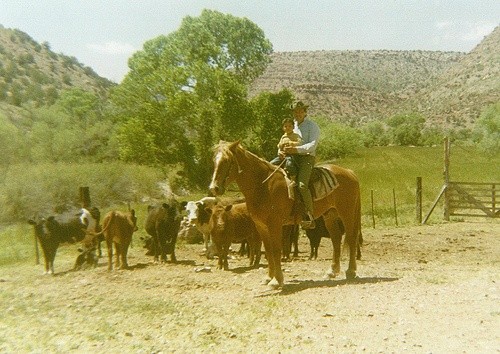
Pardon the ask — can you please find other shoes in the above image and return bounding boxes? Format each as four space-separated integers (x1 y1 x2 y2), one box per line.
290 176 297 186
302 214 315 229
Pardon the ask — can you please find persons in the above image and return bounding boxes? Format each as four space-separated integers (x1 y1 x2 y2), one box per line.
277 100 320 230
269 118 302 188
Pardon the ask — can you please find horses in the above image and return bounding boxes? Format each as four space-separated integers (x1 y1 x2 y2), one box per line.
209 138 363 286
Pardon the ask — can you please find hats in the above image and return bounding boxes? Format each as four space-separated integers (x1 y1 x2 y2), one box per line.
290 101 309 109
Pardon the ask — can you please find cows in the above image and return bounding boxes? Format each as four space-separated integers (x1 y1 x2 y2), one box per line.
282 207 363 262
84 209 138 270
185 197 232 252
27 206 100 275
204 201 262 271
146 198 188 264
